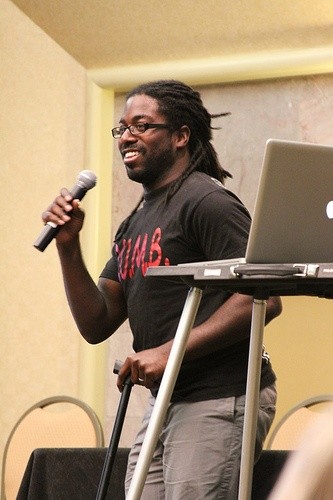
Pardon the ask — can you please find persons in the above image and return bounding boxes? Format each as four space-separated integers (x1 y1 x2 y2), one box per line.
41 80 283 500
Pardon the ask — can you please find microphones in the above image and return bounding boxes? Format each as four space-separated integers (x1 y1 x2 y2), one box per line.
34 170 96 252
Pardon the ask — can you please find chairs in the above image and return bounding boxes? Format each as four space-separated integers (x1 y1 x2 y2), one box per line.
1 396 105 500
266 394 333 449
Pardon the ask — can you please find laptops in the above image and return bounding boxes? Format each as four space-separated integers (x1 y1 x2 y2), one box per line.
178 139 333 267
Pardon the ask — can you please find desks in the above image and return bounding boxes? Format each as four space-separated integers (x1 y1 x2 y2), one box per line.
126 266 333 499
16 448 294 500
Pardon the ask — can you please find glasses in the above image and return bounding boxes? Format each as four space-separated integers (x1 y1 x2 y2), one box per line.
112 122 171 139
